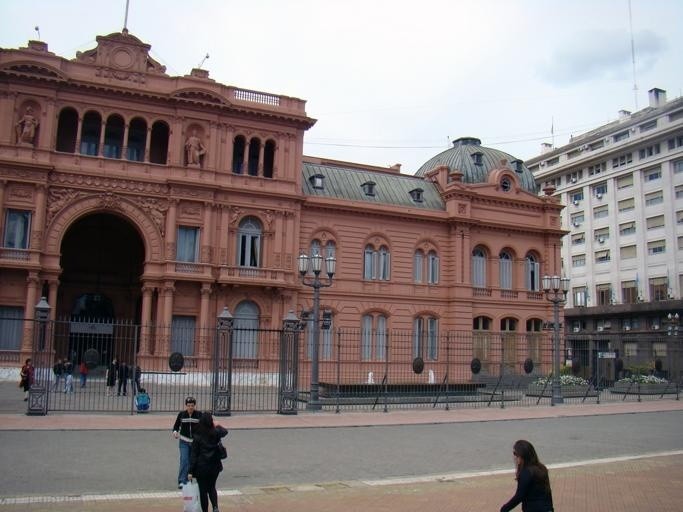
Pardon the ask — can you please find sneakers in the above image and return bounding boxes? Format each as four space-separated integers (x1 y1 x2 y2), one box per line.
213 505 219 512
178 482 184 489
23 385 140 402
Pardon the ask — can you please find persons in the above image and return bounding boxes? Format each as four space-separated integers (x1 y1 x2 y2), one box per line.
187 414 229 512
171 396 200 489
52 354 142 398
16 105 39 145
135 389 151 412
19 359 37 402
497 440 554 512
185 129 209 165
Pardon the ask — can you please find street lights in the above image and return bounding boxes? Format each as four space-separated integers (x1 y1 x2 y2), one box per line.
542 273 570 405
297 251 336 410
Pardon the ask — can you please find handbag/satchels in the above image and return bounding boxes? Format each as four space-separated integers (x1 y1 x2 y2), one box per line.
17 375 24 388
181 478 203 512
218 443 228 459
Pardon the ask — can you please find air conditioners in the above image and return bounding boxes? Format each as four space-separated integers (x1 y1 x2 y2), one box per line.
540 125 637 243
572 291 675 334
